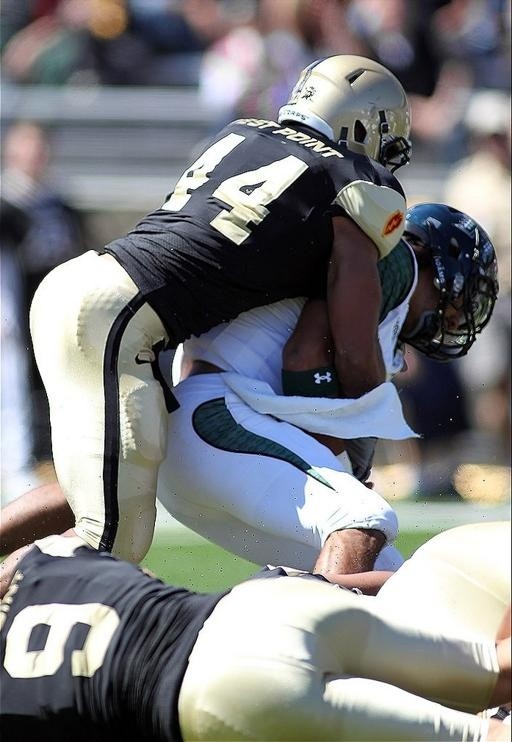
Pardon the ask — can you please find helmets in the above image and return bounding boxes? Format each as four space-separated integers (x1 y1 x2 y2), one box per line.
402 204 498 361
277 54 412 174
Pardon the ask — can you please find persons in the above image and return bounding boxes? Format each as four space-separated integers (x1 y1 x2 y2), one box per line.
0 118 84 504
152 205 487 597
0 55 410 588
0 540 511 741
445 92 509 438
1 1 511 94
